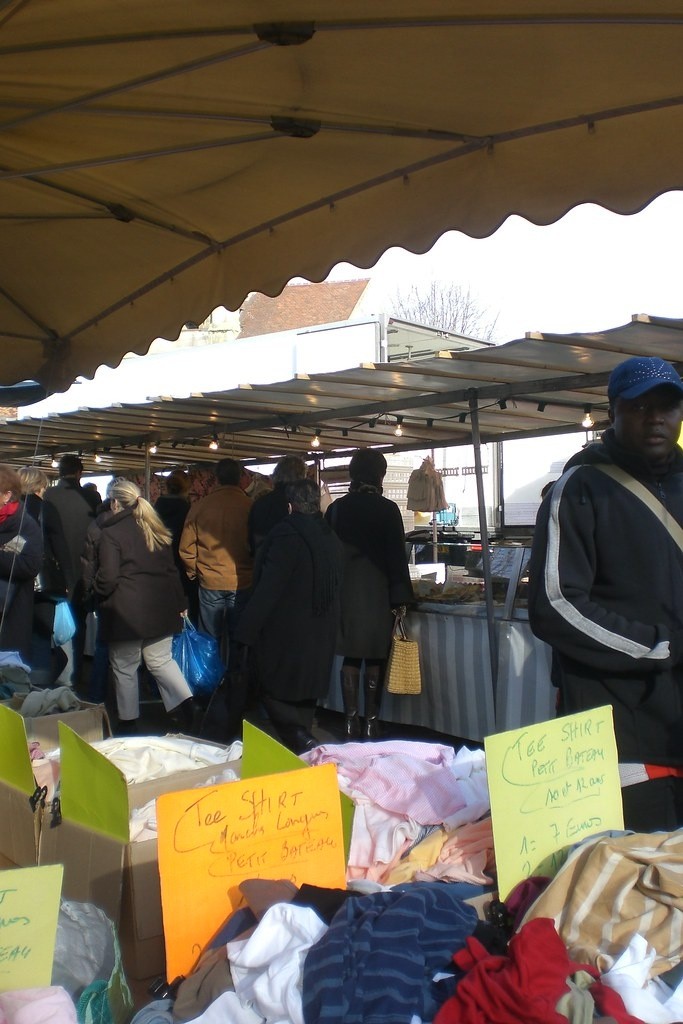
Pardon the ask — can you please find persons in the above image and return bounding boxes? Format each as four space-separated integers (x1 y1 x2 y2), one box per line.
0 448 416 756
526 356 683 834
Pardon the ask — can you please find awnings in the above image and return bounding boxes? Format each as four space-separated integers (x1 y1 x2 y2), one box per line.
0 0 683 393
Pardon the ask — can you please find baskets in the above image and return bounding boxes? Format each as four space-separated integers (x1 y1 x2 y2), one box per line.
385 612 421 695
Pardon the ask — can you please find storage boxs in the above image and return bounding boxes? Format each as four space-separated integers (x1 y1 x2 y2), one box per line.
0 692 113 753
0 732 228 868
36 757 240 982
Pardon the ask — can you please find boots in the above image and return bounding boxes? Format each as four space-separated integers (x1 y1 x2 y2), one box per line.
362 672 383 743
340 670 361 743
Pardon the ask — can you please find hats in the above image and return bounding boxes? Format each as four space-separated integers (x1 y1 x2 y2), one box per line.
607 356 683 406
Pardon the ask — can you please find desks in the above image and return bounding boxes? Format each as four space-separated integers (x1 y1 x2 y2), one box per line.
317 599 558 743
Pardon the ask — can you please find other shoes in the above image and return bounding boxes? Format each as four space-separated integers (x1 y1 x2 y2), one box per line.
112 722 137 737
183 698 202 736
307 740 323 751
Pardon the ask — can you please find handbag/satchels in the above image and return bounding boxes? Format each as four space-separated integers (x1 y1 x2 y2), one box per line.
171 612 224 698
52 597 75 647
33 548 67 602
